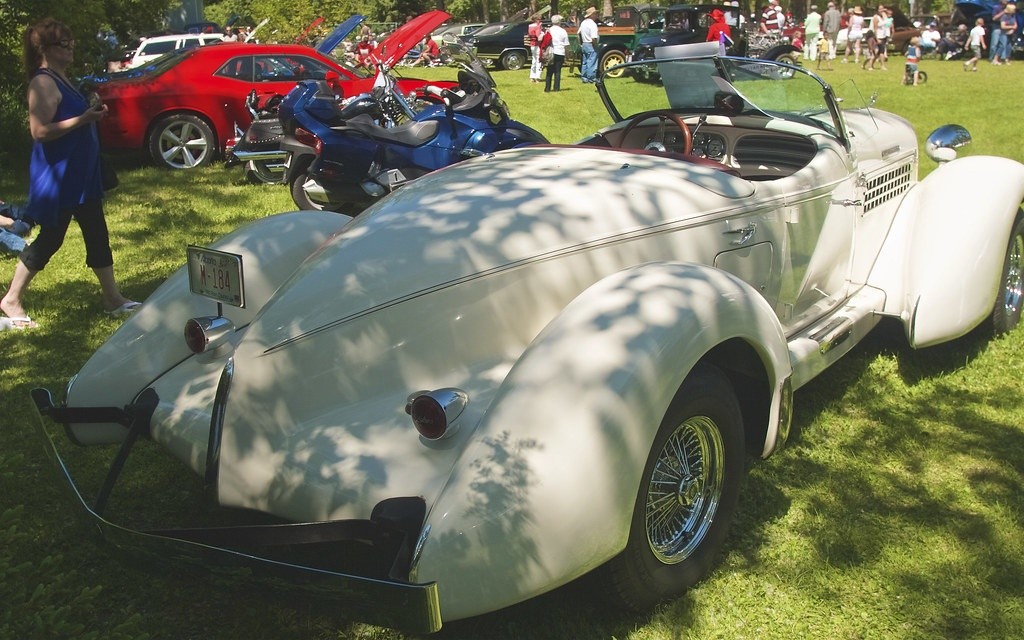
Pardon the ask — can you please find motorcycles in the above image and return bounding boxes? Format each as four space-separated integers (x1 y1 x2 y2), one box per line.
280 33 550 213
224 65 417 186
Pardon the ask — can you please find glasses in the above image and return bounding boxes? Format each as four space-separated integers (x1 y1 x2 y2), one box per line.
43 39 75 48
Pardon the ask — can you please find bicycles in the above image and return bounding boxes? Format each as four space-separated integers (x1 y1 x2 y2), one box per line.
904 64 927 86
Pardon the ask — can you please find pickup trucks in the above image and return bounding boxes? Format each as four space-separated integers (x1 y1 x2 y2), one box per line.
562 6 669 77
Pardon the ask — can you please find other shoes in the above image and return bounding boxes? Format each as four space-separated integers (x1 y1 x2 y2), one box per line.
868 67 875 71
531 78 541 83
962 63 968 72
972 67 977 71
855 60 860 64
1006 62 1011 66
842 59 848 64
992 60 1001 66
881 67 887 70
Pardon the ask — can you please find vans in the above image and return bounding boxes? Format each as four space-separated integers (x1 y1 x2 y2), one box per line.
129 34 226 70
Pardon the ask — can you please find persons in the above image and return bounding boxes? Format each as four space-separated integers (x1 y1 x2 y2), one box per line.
358 38 373 57
544 14 569 93
804 5 821 61
816 30 833 71
991 4 1017 66
578 6 599 83
568 14 576 25
930 24 968 61
989 0 1006 60
528 14 543 83
367 35 377 50
964 18 986 72
405 34 439 68
919 22 940 54
0 18 142 330
222 26 253 44
706 9 731 45
902 36 921 86
724 0 803 50
361 23 370 41
861 29 876 71
841 3 895 71
822 2 840 60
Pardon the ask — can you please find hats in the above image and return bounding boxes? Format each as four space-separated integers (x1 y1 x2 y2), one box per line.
853 6 862 14
551 14 564 24
774 6 782 11
706 9 725 23
584 6 600 19
826 2 834 7
958 24 968 30
930 22 937 27
811 5 818 9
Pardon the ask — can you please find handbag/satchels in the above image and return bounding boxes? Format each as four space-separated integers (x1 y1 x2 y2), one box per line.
100 155 119 192
524 35 532 46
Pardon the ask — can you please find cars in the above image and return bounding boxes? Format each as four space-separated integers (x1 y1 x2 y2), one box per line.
630 5 805 83
782 13 850 51
184 22 223 34
421 5 563 71
937 0 1024 61
836 6 952 53
80 9 462 171
26 43 1024 640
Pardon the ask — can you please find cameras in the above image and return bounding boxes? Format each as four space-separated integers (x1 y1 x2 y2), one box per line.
88 92 103 112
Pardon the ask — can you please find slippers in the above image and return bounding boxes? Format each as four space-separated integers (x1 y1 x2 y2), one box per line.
0 313 37 329
103 299 142 315
